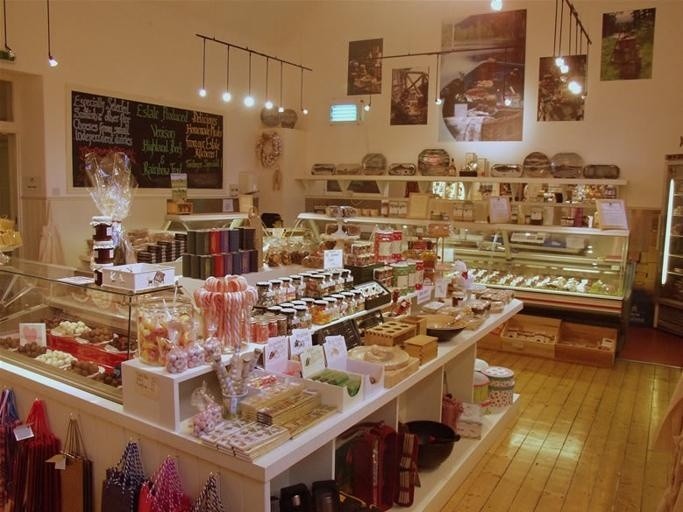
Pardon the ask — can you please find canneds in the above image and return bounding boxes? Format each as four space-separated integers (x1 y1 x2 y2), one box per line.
249 269 366 344
347 243 375 266
91 216 116 286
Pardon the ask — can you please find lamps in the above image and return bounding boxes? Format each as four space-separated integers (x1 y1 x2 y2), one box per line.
549 0 593 98
363 45 516 111
192 30 311 120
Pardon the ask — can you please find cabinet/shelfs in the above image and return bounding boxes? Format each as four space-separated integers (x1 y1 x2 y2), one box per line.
293 170 631 368
3 210 526 508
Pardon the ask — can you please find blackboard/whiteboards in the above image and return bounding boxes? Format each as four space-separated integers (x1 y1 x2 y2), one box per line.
65 86 227 193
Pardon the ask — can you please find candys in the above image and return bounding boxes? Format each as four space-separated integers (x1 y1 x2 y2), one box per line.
137 274 258 435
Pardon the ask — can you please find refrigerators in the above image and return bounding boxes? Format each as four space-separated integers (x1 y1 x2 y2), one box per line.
650 153 682 337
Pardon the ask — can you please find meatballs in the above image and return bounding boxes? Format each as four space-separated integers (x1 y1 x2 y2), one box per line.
0 316 137 388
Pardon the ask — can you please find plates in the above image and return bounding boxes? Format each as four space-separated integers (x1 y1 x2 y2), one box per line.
312 150 450 176
0 319 135 378
491 152 620 177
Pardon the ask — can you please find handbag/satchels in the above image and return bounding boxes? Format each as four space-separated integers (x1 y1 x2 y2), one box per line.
0 418 190 512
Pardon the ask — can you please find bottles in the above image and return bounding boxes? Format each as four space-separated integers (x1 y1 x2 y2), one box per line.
253 269 365 343
136 296 192 367
373 228 425 295
450 158 456 177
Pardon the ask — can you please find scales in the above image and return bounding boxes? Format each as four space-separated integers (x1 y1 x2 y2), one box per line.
510 232 546 244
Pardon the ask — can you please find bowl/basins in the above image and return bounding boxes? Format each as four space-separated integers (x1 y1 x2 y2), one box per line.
420 313 468 342
407 420 456 470
